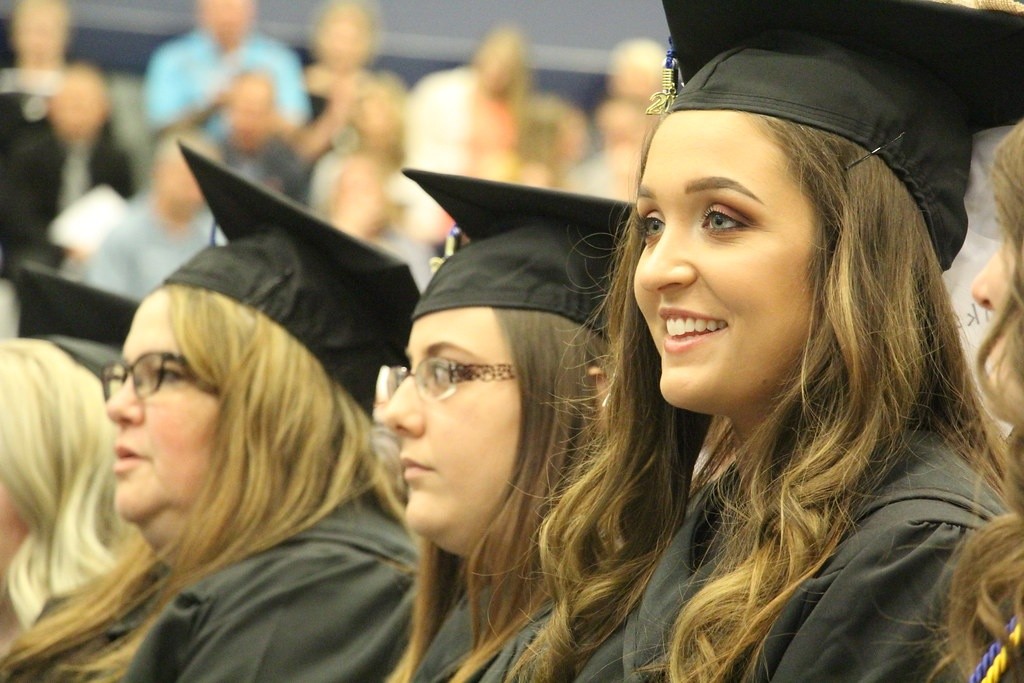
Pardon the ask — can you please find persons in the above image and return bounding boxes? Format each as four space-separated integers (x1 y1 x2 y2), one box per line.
943 120 1024 683
0 138 422 683
376 167 635 683
0 0 672 304
0 261 140 654
503 0 1024 683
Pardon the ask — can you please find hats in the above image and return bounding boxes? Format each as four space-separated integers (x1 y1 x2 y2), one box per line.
166 139 419 421
13 261 138 350
647 0 1024 276
404 167 665 370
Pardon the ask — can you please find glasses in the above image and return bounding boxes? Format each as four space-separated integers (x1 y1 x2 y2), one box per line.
101 352 223 402
376 357 521 406
